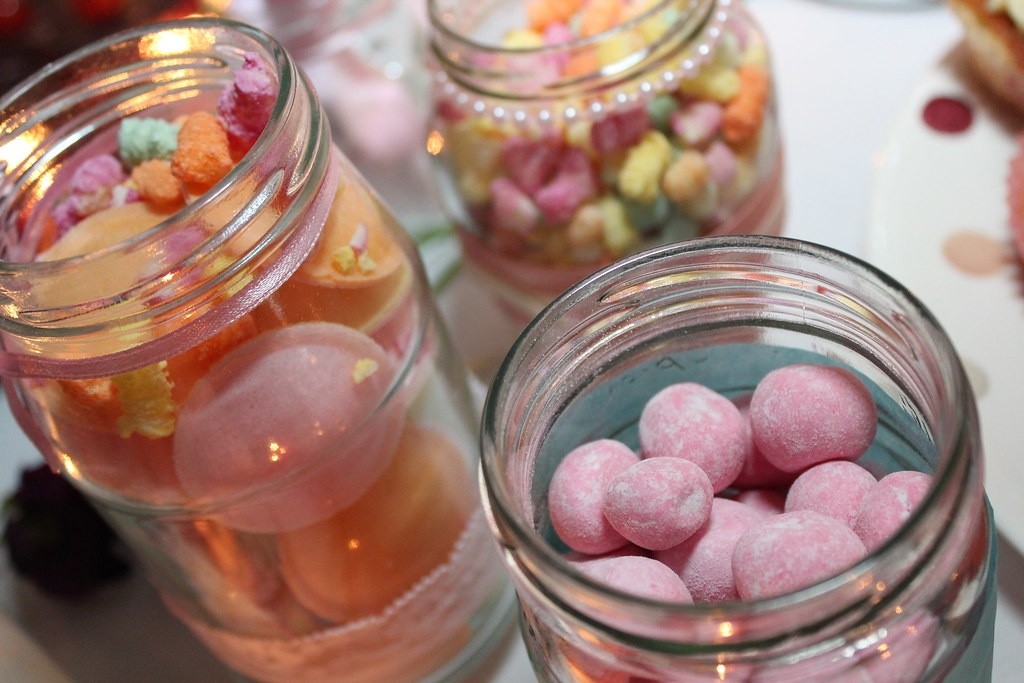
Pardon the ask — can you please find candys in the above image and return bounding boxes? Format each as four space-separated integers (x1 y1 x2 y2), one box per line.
0 0 985 682
